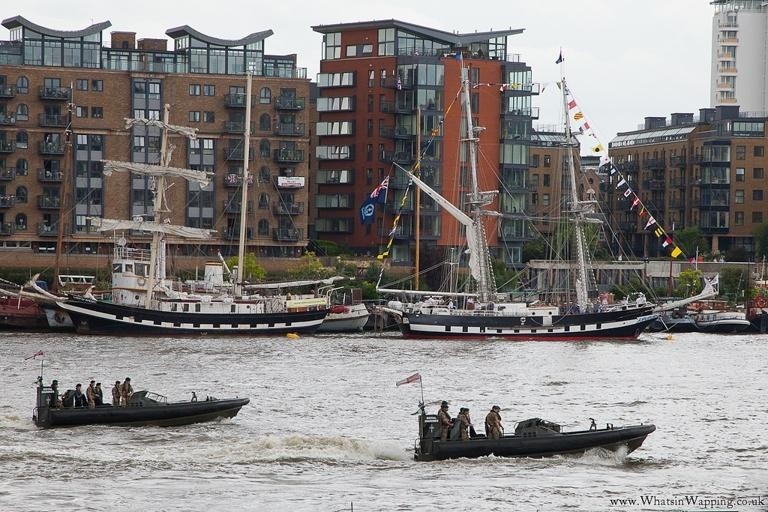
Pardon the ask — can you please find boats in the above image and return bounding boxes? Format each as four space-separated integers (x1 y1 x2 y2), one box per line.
408 375 659 461
31 353 252 430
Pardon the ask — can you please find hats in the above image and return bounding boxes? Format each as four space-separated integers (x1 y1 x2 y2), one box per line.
76 384 81 387
463 409 468 412
126 378 130 381
116 381 119 384
443 405 448 408
493 406 500 409
53 380 57 383
91 381 95 383
442 401 447 404
96 383 100 386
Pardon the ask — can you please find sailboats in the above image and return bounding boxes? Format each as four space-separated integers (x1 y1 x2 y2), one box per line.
0 42 767 344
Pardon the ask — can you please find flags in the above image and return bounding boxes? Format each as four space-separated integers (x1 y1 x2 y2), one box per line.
359 174 388 226
472 82 549 94
396 372 420 386
557 76 682 261
555 53 565 64
33 349 44 360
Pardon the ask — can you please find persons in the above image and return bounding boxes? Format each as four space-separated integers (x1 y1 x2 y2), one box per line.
47 379 65 408
112 381 123 406
484 404 505 439
460 406 472 439
457 407 464 418
94 381 103 404
439 405 454 440
437 401 448 420
75 384 87 407
86 380 99 407
120 377 134 406
